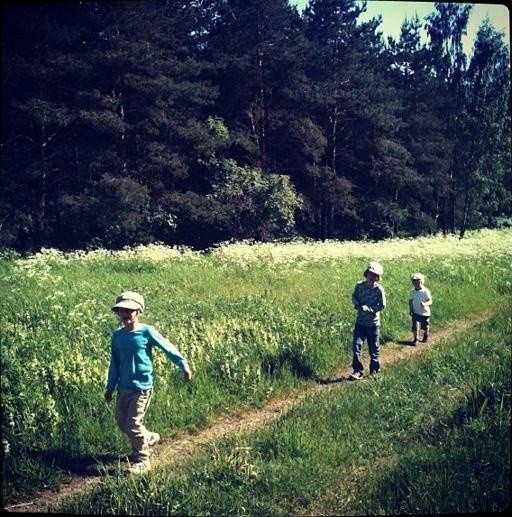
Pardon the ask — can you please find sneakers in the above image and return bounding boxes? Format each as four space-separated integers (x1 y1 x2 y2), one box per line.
349 372 381 380
126 432 161 475
411 334 429 346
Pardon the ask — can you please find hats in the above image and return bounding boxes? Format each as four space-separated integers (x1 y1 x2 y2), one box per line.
111 291 146 315
368 261 383 275
412 273 424 280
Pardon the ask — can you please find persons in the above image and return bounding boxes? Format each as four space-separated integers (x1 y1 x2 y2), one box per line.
103 291 193 475
348 261 386 381
406 271 434 346
